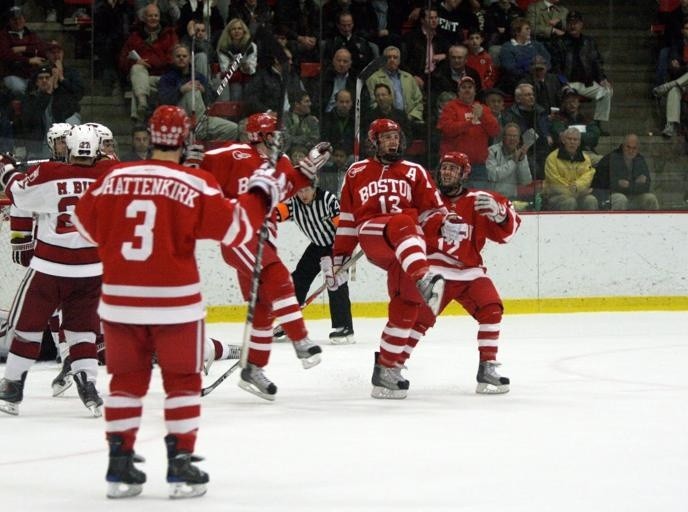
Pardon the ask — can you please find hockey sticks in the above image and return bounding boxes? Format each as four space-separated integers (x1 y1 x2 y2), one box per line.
239 65 285 368
200 249 364 395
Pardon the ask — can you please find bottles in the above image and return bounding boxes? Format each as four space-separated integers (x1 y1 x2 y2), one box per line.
534 192 542 212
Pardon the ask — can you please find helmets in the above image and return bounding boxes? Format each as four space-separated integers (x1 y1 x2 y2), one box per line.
245 112 278 144
436 150 471 193
47 122 115 159
149 104 189 146
368 118 406 161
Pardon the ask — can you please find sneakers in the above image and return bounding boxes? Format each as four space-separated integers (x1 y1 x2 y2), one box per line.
371 351 410 391
271 325 286 338
292 336 321 359
226 345 245 360
240 362 278 395
476 360 510 387
416 271 445 314
593 122 610 137
50 356 104 408
329 323 354 339
106 433 147 486
653 81 675 98
662 121 676 137
163 433 210 485
0 371 27 404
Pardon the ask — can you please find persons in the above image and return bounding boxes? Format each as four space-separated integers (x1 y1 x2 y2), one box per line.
271 177 354 339
1 121 75 395
325 118 471 390
372 152 509 390
0 0 687 213
56 121 119 368
72 104 254 482
183 111 333 393
0 124 120 405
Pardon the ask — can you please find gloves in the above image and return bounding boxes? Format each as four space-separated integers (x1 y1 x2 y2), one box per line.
319 254 353 292
0 151 21 193
247 161 288 219
474 193 507 223
441 212 470 242
182 144 205 169
298 141 333 181
10 234 34 266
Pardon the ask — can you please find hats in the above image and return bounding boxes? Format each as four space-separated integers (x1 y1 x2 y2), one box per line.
37 65 54 76
566 11 582 21
457 76 475 88
9 5 22 19
44 39 60 49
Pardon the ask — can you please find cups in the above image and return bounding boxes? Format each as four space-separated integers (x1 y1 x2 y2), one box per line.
550 106 560 119
